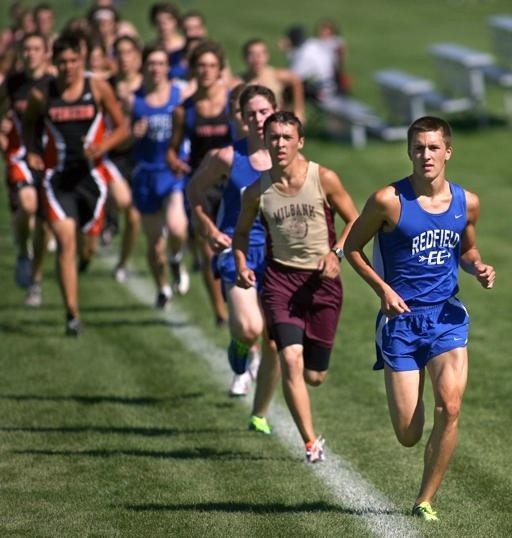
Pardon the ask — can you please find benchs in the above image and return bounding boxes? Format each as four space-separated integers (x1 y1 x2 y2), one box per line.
426 41 496 104
324 95 385 147
370 68 434 124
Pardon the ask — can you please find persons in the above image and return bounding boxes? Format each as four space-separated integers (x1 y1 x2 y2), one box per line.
187 86 304 434
0 1 355 335
191 84 265 379
231 110 360 466
344 118 498 521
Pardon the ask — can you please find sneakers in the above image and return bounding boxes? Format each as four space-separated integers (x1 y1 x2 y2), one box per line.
227 336 248 376
154 292 169 308
411 501 440 522
15 253 34 287
305 440 324 464
23 283 43 307
248 415 273 435
168 258 190 297
245 344 262 380
110 265 128 283
229 369 252 396
65 318 82 336
